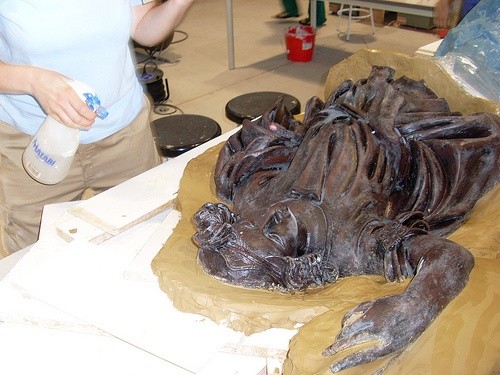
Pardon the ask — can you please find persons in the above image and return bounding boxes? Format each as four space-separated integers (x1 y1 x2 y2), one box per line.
1 0 196 260
276 0 327 26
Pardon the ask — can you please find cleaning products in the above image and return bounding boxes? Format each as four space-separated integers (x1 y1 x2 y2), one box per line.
21 79 109 185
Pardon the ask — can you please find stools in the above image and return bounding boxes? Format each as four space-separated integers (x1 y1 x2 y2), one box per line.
226 91 300 124
150 114 221 157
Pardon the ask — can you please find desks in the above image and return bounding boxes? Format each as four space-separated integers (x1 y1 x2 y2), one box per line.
0 117 500 374
